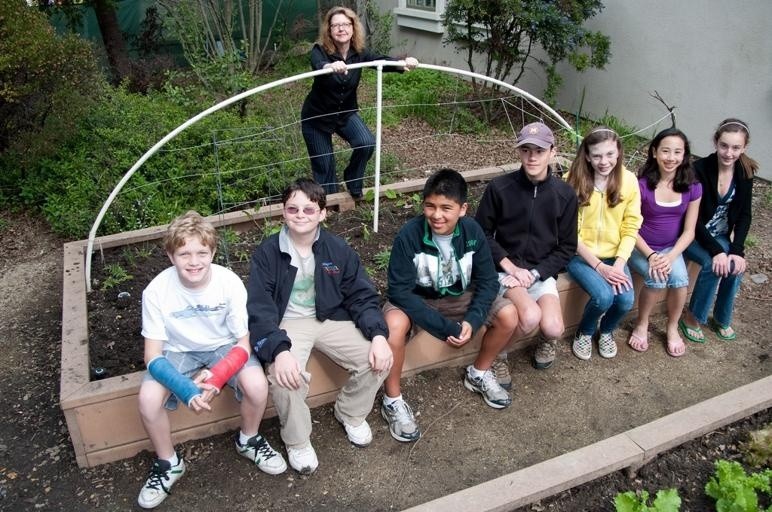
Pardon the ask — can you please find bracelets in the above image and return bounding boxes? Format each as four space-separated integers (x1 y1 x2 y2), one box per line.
647 251 656 261
594 262 602 271
530 268 539 281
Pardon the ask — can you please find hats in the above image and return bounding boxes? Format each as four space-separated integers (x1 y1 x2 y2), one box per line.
513 122 554 150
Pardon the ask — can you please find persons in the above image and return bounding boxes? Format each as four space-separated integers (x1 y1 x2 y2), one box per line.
301 6 419 200
382 169 519 443
137 210 288 509
679 117 760 342
627 127 702 357
246 177 394 475
561 124 644 359
474 122 578 391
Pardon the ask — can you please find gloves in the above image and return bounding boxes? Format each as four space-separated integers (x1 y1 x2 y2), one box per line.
203 346 249 396
147 355 203 408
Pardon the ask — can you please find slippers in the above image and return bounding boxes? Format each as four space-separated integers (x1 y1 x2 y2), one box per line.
628 333 649 351
666 337 686 356
679 319 706 343
708 317 736 340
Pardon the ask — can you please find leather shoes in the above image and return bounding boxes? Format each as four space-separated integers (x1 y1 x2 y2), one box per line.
344 169 362 199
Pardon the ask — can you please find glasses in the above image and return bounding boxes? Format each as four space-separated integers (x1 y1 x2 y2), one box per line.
331 22 353 29
284 203 321 215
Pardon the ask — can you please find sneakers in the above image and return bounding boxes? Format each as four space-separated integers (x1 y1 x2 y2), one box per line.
464 364 511 409
138 452 185 509
334 409 373 447
490 353 512 391
572 326 592 360
380 395 420 443
597 324 617 359
533 329 557 369
235 429 287 476
287 437 319 474
